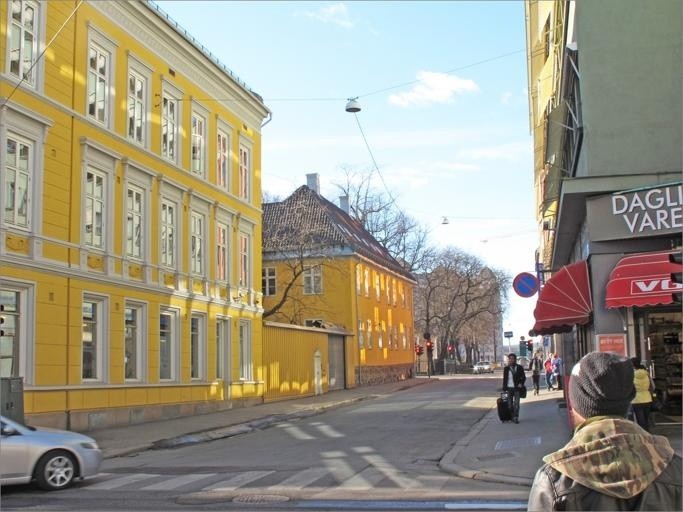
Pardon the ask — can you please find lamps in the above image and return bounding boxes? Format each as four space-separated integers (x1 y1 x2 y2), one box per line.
442 217 449 224
345 95 362 112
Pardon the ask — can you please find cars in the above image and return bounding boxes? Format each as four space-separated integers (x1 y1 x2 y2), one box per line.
523 362 528 371
0 413 106 493
471 361 494 374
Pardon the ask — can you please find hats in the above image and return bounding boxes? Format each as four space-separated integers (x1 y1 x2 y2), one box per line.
568 351 637 421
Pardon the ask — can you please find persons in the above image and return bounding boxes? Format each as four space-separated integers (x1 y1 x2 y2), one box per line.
502 354 527 424
527 349 543 396
551 353 563 391
629 356 656 432
544 353 552 390
528 350 683 512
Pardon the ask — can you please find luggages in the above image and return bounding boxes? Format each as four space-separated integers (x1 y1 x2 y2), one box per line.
497 392 511 423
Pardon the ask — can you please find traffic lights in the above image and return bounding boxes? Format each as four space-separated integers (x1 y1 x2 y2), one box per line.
448 346 451 352
419 346 423 356
430 342 433 351
425 342 430 353
415 345 420 355
527 339 532 351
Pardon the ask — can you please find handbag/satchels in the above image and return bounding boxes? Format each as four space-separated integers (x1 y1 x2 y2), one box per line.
520 387 526 398
